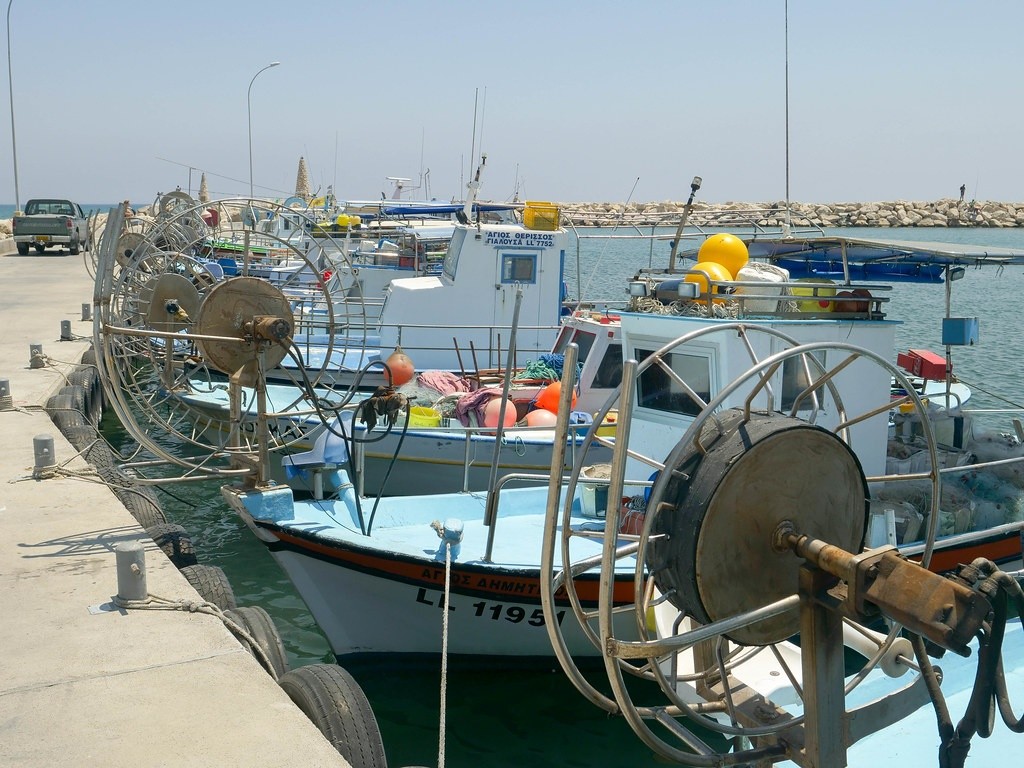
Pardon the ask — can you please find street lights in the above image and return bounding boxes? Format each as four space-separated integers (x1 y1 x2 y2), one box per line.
247 61 284 207
187 166 197 196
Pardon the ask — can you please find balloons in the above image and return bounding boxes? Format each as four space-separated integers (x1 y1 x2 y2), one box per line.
337 213 362 227
686 230 751 306
481 378 578 431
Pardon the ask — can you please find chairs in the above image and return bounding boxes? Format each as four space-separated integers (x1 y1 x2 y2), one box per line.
282 410 353 500
202 263 224 282
217 258 237 276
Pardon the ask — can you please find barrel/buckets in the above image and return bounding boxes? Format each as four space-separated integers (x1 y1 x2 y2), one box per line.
408 406 441 427
568 411 593 437
594 408 619 437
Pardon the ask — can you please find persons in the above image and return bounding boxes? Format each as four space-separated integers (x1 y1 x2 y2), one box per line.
959 184 966 202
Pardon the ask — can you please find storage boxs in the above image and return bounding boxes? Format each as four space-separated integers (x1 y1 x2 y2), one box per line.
356 239 419 267
618 497 645 536
524 201 559 230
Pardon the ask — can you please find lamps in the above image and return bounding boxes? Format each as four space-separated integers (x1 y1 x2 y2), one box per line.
691 176 702 194
629 282 651 297
950 267 965 281
678 282 700 299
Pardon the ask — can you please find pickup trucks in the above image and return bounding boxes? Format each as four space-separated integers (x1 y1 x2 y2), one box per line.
12 199 93 256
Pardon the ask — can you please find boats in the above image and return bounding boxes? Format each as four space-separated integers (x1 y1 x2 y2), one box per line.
536 319 1023 768
81 0 1023 660
85 169 626 387
